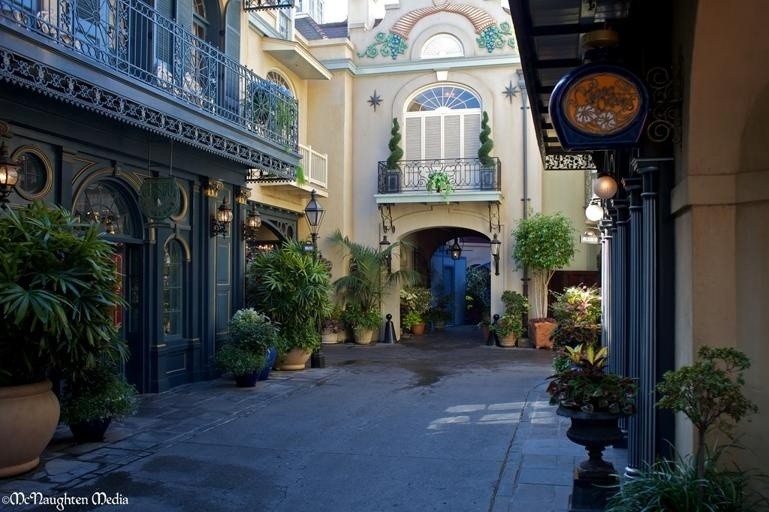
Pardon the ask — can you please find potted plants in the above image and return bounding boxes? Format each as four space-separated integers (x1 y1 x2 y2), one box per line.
215 341 269 388
322 227 452 345
229 306 282 381
510 207 583 350
57 371 143 438
488 289 532 348
546 357 642 471
0 197 132 478
246 234 336 371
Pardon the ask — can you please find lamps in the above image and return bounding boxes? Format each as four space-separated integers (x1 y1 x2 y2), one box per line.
210 195 233 240
379 236 391 275
490 232 501 276
448 237 463 260
0 140 23 203
592 170 635 200
585 200 605 222
241 201 263 243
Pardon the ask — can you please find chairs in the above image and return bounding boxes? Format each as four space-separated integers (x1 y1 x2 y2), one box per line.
156 65 216 115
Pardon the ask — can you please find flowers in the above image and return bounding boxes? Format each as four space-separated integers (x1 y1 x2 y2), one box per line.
547 281 608 367
